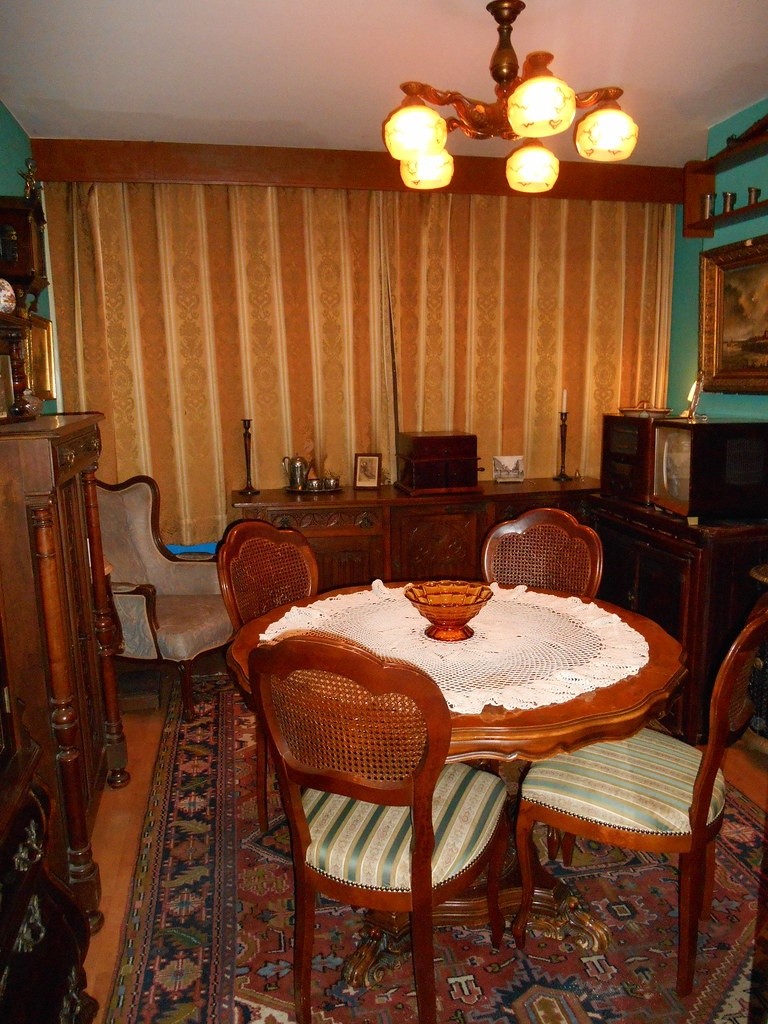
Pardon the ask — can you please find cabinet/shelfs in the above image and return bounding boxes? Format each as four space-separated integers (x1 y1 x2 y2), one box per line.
0 412 133 934
601 408 652 504
682 127 768 239
232 473 601 583
576 493 768 745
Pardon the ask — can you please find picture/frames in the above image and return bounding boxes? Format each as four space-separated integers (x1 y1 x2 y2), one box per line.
698 232 768 395
352 452 384 490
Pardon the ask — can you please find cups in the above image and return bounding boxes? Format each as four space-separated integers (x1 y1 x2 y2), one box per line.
307 478 339 490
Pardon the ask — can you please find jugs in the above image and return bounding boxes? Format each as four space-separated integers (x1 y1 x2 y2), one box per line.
282 452 313 490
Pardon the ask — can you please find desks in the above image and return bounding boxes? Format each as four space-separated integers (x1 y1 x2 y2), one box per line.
223 580 690 940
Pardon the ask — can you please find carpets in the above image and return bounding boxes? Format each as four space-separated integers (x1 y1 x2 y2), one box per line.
103 668 768 1024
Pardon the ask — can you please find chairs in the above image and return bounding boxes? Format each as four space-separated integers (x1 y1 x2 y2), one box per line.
218 521 319 836
480 506 605 597
247 631 511 1024
82 475 232 723
509 589 768 998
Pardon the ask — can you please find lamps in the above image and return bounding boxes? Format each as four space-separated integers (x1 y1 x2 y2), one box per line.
385 0 639 193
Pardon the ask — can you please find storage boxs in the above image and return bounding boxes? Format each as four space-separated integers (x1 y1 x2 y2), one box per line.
394 429 479 460
395 455 445 488
446 459 478 486
118 669 162 713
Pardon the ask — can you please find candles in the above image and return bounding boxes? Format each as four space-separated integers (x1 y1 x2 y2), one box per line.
561 387 567 412
